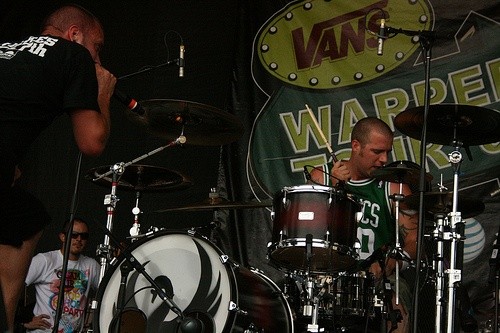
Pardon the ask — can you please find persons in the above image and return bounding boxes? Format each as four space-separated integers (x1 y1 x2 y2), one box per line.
14 217 101 333
0 5 119 333
248 116 417 333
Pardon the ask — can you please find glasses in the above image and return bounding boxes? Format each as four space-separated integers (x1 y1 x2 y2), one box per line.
68 230 91 243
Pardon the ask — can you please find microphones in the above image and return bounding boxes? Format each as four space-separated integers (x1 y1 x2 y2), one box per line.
179 41 185 77
362 244 389 270
113 87 145 116
377 13 385 55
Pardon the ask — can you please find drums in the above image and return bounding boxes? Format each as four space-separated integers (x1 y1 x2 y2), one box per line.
335 271 376 319
269 185 359 272
92 229 295 333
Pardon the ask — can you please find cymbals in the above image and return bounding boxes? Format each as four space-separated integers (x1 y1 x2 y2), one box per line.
399 192 485 221
154 202 272 213
125 99 244 146
393 105 500 146
370 167 434 184
84 164 194 194
